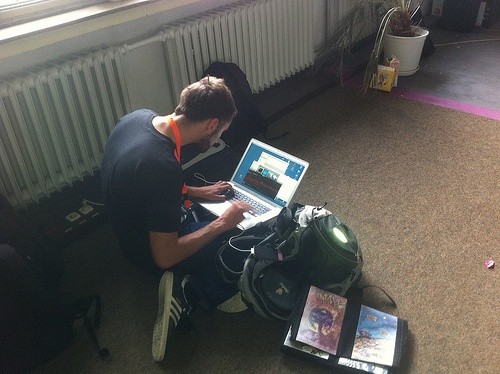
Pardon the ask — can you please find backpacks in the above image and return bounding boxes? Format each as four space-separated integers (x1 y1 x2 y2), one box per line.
203 63 266 147
238 204 364 320
0 244 74 374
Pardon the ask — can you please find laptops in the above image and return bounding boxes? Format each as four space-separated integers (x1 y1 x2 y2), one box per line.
192 138 310 233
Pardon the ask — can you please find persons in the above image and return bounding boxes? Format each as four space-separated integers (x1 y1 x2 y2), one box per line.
100 75 248 361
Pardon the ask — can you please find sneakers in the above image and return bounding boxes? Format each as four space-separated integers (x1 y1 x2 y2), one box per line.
151 272 192 361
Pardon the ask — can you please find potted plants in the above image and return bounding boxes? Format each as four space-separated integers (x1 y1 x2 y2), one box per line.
314 0 431 97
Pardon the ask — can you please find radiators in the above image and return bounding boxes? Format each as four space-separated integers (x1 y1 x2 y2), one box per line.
0 0 424 214
